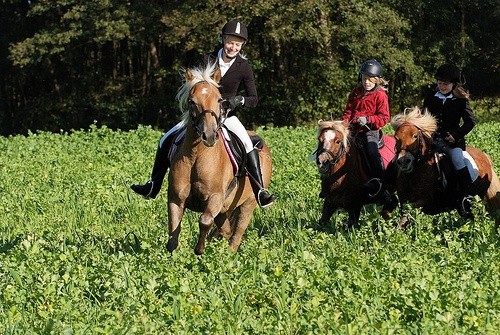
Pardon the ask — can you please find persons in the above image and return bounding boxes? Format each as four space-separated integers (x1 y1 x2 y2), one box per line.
130 21 276 207
421 63 476 197
340 59 395 209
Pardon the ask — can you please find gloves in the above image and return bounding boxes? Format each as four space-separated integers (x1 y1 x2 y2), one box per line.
357 116 368 126
226 95 244 111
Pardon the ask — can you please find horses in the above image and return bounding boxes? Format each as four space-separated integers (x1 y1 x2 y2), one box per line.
314 119 397 232
166 56 271 255
387 106 500 222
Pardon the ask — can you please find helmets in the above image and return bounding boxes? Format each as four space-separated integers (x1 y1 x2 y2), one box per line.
434 63 460 84
222 21 248 41
360 60 383 78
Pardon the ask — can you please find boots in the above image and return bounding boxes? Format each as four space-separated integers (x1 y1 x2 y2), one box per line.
130 144 169 199
456 166 473 213
364 141 383 196
246 148 276 206
